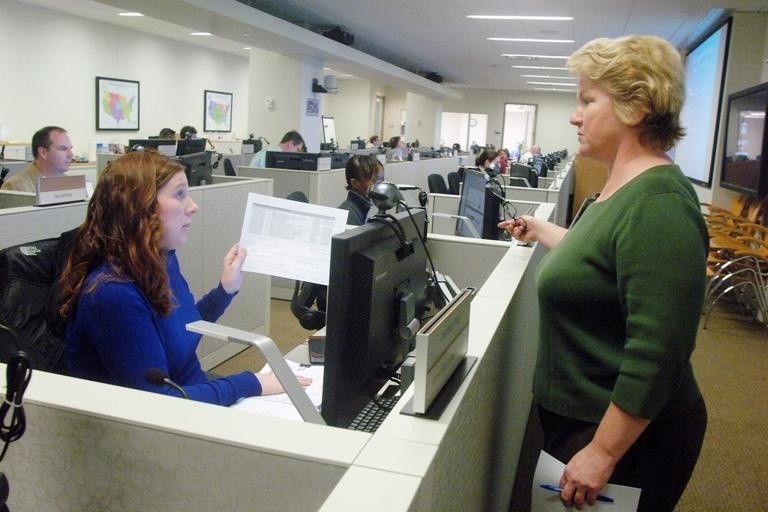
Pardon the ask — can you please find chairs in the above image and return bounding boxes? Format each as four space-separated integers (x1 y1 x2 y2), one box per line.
698 190 767 330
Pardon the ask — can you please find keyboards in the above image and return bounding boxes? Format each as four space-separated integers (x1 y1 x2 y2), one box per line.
348 394 399 433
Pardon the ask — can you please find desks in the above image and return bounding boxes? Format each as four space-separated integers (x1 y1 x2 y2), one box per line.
2 123 579 511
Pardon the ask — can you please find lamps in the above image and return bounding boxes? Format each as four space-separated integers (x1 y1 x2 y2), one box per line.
311 70 338 95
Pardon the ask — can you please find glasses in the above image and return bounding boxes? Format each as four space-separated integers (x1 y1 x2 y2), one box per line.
503 199 529 231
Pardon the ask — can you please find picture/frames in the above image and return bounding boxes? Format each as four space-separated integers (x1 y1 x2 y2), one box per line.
204 90 233 133
306 99 321 117
96 75 140 131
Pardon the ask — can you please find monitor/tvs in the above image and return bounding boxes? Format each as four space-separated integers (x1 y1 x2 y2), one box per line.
321 207 427 427
128 138 177 157
244 140 262 153
320 143 332 150
455 168 503 240
720 82 767 197
318 152 350 169
177 151 212 187
175 138 207 156
351 140 365 149
509 162 538 188
265 151 321 172
533 149 568 177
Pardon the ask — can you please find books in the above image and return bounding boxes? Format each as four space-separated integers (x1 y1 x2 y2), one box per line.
4 141 34 162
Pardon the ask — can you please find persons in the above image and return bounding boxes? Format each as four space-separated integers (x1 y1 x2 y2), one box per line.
158 128 175 139
365 135 380 148
53 153 320 406
476 141 543 173
389 136 406 159
1 127 75 196
494 30 714 511
180 126 196 139
334 153 385 224
250 130 305 167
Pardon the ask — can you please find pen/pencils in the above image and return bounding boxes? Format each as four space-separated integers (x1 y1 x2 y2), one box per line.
540 484 615 502
